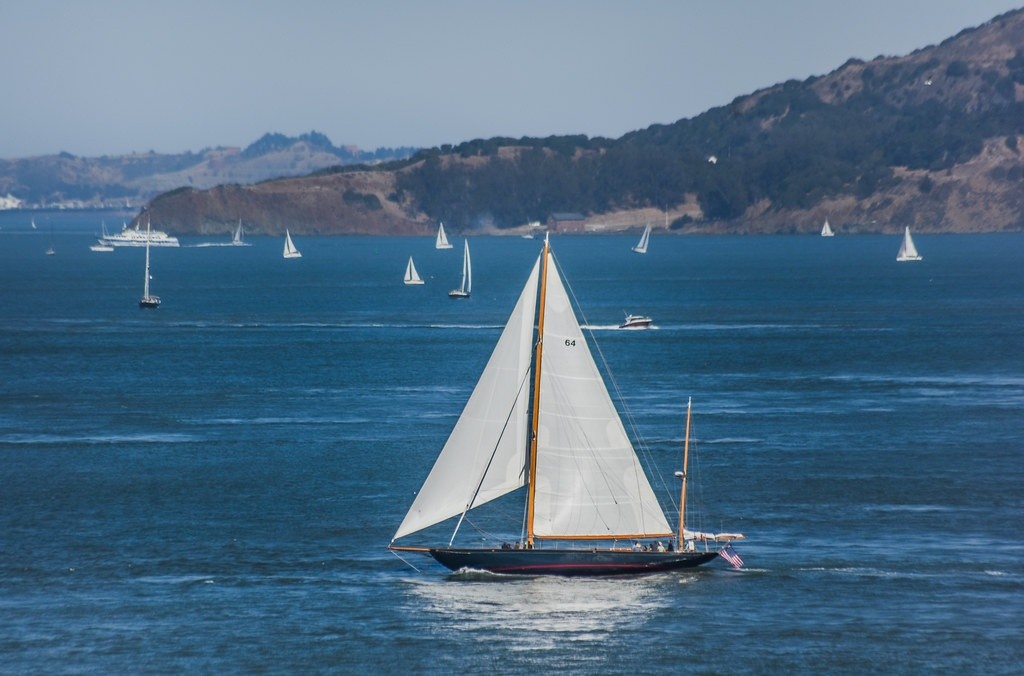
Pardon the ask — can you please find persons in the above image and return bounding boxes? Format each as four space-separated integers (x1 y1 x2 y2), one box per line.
657 542 665 552
666 539 674 550
514 542 519 550
506 544 512 549
527 541 531 548
633 541 657 551
502 541 508 549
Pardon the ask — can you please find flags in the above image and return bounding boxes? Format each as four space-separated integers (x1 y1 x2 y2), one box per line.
719 543 744 569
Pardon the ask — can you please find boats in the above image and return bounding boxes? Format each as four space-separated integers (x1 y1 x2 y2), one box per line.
619 313 652 330
89 246 114 252
46 249 55 255
99 222 180 247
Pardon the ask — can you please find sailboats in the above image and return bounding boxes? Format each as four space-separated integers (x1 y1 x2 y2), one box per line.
387 227 746 578
283 228 303 259
435 222 453 249
447 236 473 297
820 219 835 237
631 223 652 254
230 217 253 247
138 215 162 309
896 225 923 262
403 258 425 285
